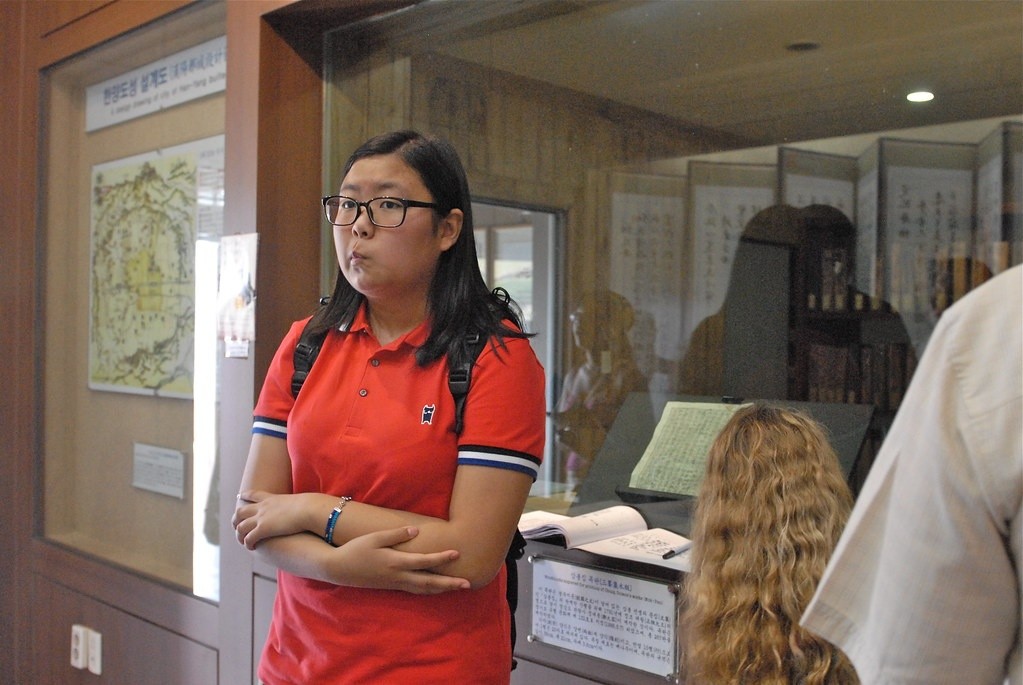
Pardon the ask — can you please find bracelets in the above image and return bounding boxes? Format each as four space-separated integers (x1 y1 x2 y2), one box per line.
323 495 352 548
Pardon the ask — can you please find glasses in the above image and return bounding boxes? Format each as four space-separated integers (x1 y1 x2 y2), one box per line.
569 311 584 322
321 196 437 228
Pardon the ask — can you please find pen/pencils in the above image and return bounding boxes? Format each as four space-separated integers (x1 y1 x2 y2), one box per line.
662 540 694 560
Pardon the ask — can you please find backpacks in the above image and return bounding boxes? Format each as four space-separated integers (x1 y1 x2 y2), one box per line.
290 277 532 678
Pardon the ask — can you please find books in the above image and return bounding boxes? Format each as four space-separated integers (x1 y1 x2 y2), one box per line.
516 506 692 575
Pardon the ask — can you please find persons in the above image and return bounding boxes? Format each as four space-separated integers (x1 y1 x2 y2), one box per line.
552 288 647 484
231 129 546 684
682 404 860 684
679 205 920 498
799 265 1023 685
